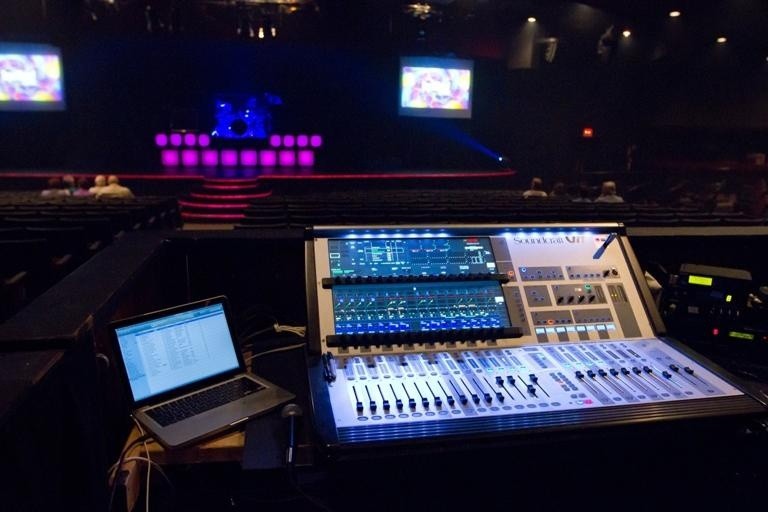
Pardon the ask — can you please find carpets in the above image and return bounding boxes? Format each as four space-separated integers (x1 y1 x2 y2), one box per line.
0 219 176 424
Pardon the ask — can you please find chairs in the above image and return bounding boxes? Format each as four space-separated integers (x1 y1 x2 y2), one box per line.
0 191 179 287
234 190 768 231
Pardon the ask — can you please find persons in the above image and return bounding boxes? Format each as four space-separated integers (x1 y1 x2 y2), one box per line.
89 176 107 195
63 176 76 197
103 176 131 196
73 177 91 197
594 182 623 203
40 179 69 198
524 177 547 197
573 187 591 202
550 183 569 196
710 181 738 210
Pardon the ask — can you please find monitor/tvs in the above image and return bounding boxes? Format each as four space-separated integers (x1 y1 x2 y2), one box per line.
0 42 65 111
401 54 468 118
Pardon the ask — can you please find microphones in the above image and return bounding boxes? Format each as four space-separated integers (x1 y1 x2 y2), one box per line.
280 403 304 474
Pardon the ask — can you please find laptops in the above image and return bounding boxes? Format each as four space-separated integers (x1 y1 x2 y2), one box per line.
106 293 298 453
